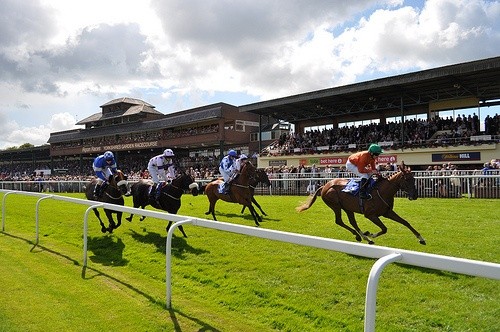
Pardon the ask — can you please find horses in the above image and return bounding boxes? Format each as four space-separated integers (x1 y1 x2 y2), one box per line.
296 165 427 246
198 160 272 226
85 176 128 234
124 171 199 238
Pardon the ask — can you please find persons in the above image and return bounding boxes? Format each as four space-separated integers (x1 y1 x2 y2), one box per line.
482 158 500 186
375 161 407 171
264 164 346 187
423 162 460 193
345 144 383 199
262 112 500 157
219 150 237 192
0 124 223 192
93 151 118 194
147 149 175 200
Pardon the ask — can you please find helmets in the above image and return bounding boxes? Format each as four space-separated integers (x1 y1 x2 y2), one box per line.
103 151 114 160
227 150 236 157
238 154 248 160
163 149 174 157
368 144 384 154
